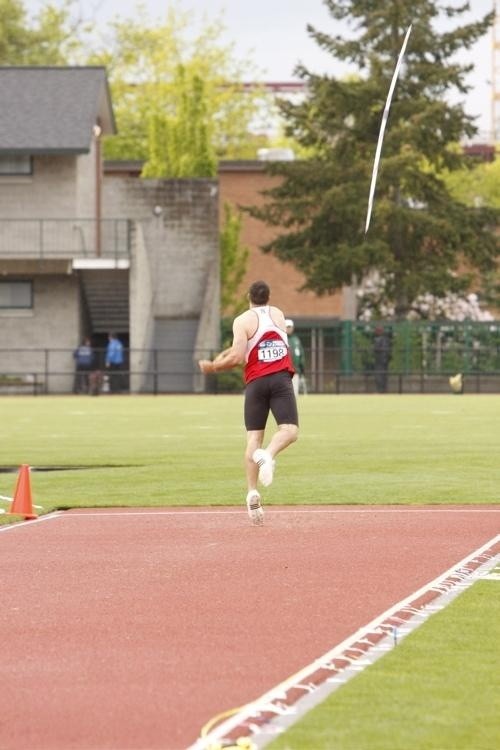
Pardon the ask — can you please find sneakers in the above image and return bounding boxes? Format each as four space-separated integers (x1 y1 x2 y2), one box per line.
253 449 274 487
246 490 264 526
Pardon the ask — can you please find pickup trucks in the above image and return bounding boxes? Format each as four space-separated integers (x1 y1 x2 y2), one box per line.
354 299 500 368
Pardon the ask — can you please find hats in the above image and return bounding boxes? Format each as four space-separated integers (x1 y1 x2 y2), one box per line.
285 319 293 327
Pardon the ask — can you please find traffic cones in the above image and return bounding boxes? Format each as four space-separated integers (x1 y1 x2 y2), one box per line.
4 460 41 523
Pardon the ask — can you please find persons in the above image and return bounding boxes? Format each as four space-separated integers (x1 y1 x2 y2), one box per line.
370 328 395 392
105 331 131 393
198 277 301 520
73 337 97 394
285 317 308 396
